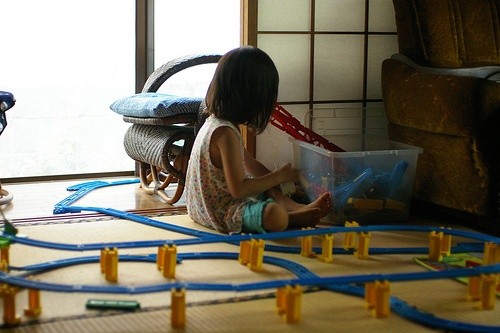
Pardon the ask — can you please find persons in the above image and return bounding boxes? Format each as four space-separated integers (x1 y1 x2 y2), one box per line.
184 47 333 235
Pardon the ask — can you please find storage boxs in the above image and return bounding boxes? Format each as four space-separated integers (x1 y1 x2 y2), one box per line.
291 139 418 225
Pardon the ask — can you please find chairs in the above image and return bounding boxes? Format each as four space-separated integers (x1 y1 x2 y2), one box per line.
110 55 220 204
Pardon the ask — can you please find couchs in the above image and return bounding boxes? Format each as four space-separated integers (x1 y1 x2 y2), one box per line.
380 0 500 236
